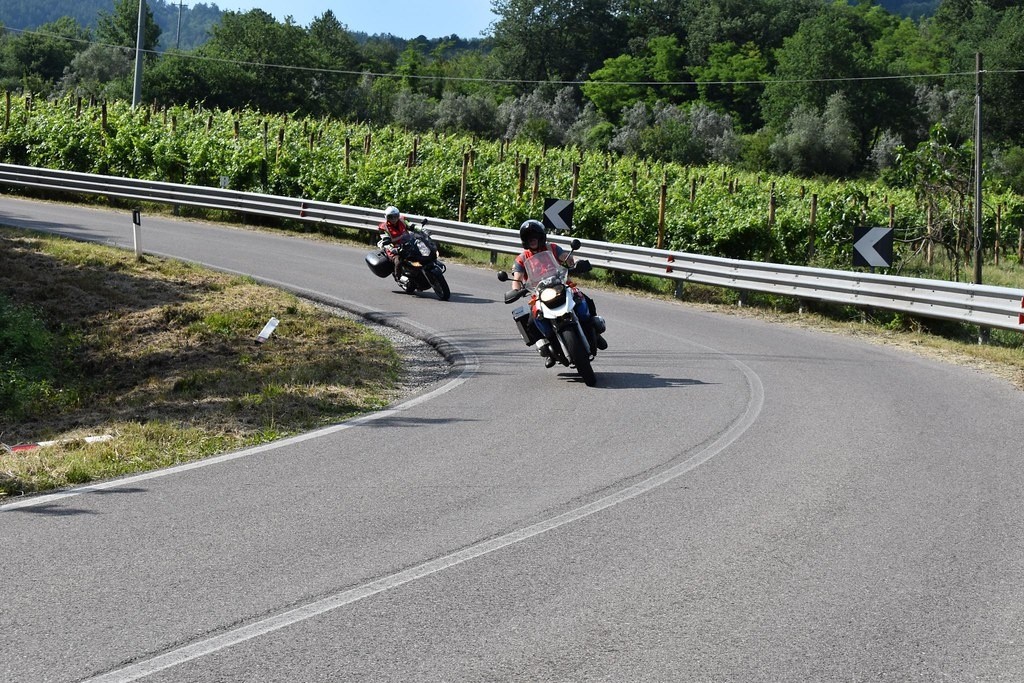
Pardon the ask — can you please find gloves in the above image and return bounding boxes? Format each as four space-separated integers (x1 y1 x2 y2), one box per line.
574 260 593 273
504 289 520 304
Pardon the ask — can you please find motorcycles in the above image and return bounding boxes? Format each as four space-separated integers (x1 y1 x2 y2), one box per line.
364 218 451 301
496 239 598 388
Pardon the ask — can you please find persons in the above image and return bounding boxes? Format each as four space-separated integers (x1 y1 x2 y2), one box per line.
511 220 608 367
376 205 431 288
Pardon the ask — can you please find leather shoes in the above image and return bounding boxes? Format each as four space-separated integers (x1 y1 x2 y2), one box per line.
545 357 555 368
597 334 608 350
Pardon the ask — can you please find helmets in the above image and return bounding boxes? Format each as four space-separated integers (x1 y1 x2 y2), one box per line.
520 220 546 249
384 206 400 222
